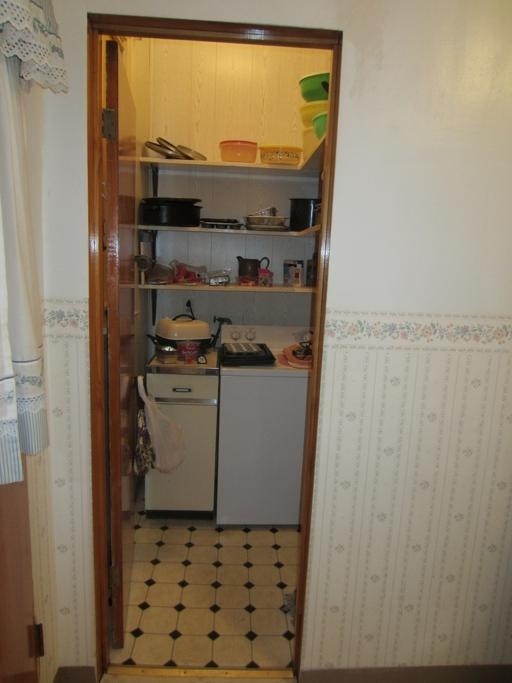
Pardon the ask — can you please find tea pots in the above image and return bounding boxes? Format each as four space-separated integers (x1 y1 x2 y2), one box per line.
236 255 270 280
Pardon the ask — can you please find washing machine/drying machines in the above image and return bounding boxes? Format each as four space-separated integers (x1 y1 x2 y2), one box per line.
215 323 311 525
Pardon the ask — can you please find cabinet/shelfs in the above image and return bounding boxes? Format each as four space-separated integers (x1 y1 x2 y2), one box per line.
132 134 324 292
139 343 219 523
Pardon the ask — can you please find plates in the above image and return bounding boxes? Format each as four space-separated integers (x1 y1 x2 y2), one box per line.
201 217 244 230
141 197 203 204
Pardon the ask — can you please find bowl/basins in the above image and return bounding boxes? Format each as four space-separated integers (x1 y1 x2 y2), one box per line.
138 203 204 227
